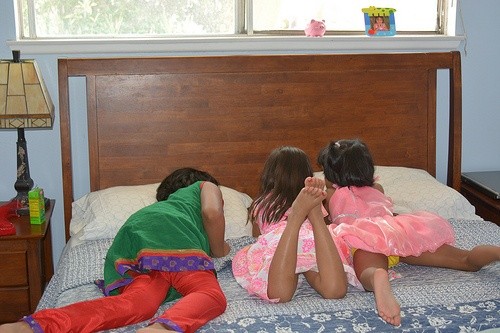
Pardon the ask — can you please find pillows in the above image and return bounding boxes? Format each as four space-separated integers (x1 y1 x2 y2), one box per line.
315 166 485 223
68 183 256 240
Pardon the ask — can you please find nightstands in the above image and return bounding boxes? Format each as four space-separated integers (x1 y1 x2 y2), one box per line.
459 170 500 228
0 198 56 323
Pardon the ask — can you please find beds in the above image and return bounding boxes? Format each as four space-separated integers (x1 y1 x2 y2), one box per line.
34 51 500 333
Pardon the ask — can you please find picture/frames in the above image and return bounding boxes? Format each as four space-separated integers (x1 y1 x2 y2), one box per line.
362 7 396 36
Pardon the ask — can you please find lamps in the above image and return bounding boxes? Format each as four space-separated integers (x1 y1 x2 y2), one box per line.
0 50 56 216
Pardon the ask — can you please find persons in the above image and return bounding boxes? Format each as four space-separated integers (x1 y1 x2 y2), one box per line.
373 16 388 31
232 146 364 304
317 137 500 325
0 167 230 333
